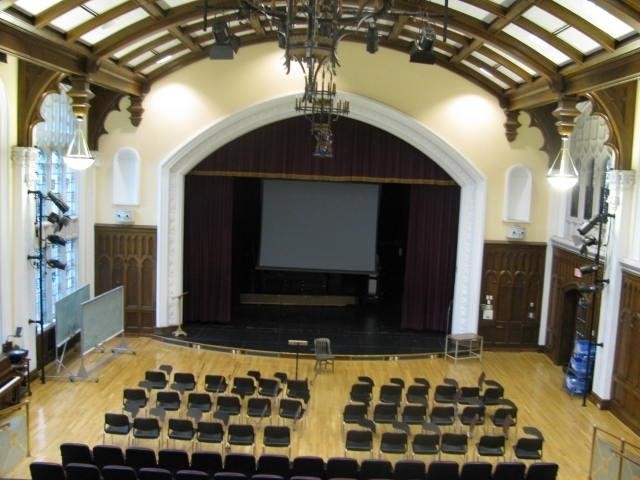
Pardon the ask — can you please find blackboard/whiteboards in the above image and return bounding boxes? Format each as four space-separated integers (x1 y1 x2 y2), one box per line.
55 284 90 348
80 285 124 356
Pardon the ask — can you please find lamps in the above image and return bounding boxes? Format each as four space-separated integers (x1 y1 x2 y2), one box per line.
508 227 526 241
573 187 616 405
115 209 133 224
545 135 579 192
28 189 73 383
61 116 96 171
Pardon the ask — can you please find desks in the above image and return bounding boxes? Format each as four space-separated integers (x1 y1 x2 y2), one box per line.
444 333 484 368
